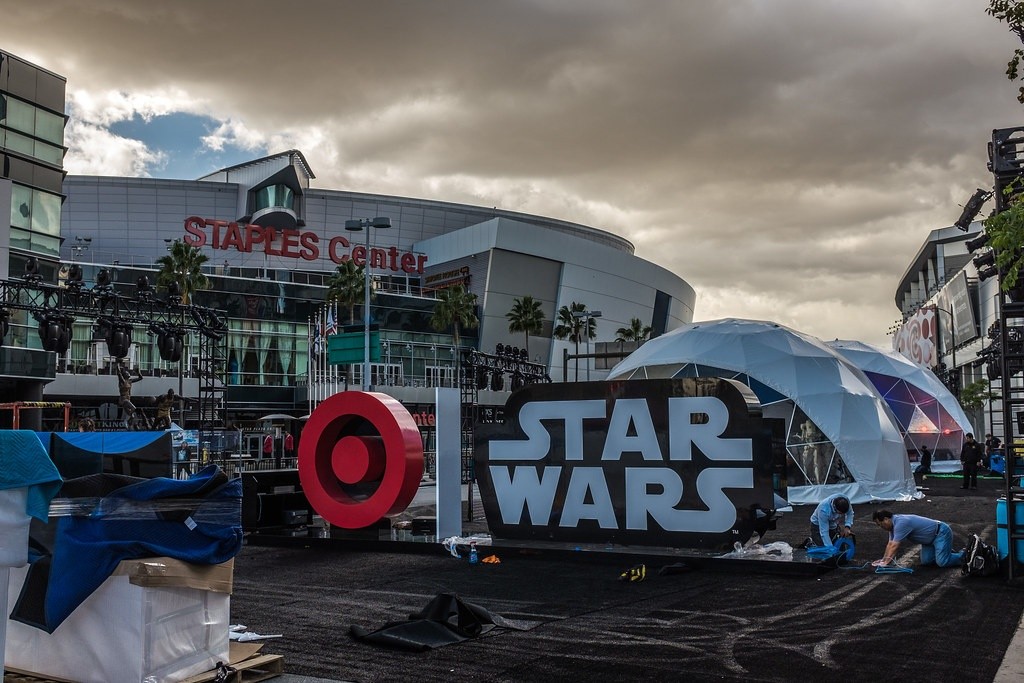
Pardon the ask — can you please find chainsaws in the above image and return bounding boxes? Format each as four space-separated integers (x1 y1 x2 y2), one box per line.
831 532 856 564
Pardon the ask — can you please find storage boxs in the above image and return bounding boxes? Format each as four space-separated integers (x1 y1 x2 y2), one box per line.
285 509 308 524
412 516 436 535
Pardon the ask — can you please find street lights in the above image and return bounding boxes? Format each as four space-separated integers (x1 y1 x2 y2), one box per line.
345 216 392 390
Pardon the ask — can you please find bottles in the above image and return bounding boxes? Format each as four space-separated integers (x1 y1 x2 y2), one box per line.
469 544 477 563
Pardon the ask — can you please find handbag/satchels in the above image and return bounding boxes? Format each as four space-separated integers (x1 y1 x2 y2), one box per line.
959 533 1000 581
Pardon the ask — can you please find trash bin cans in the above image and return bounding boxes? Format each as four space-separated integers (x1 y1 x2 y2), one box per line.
991 448 1006 475
995 490 1024 564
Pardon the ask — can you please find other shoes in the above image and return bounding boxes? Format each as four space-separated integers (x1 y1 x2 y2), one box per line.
959 485 969 489
799 536 814 550
971 486 978 491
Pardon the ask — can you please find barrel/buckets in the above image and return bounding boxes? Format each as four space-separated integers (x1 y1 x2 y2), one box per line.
991 454 1003 474
996 500 1024 562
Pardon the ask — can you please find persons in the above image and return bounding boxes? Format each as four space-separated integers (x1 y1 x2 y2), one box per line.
176 441 192 480
78 417 96 432
915 445 931 473
980 434 1001 470
960 433 982 490
284 430 294 469
263 431 273 463
116 362 143 432
151 388 199 429
799 493 856 551
871 510 966 567
223 260 229 276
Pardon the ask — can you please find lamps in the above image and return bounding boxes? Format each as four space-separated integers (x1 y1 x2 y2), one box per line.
965 234 992 254
953 187 995 234
977 265 998 283
0 257 185 364
972 249 995 270
475 342 555 394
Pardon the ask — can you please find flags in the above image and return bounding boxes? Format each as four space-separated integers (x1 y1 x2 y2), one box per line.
308 303 338 361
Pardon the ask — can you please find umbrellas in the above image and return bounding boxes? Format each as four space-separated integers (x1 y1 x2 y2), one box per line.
257 414 316 420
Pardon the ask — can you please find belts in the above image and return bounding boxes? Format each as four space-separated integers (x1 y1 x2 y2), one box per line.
930 524 941 547
